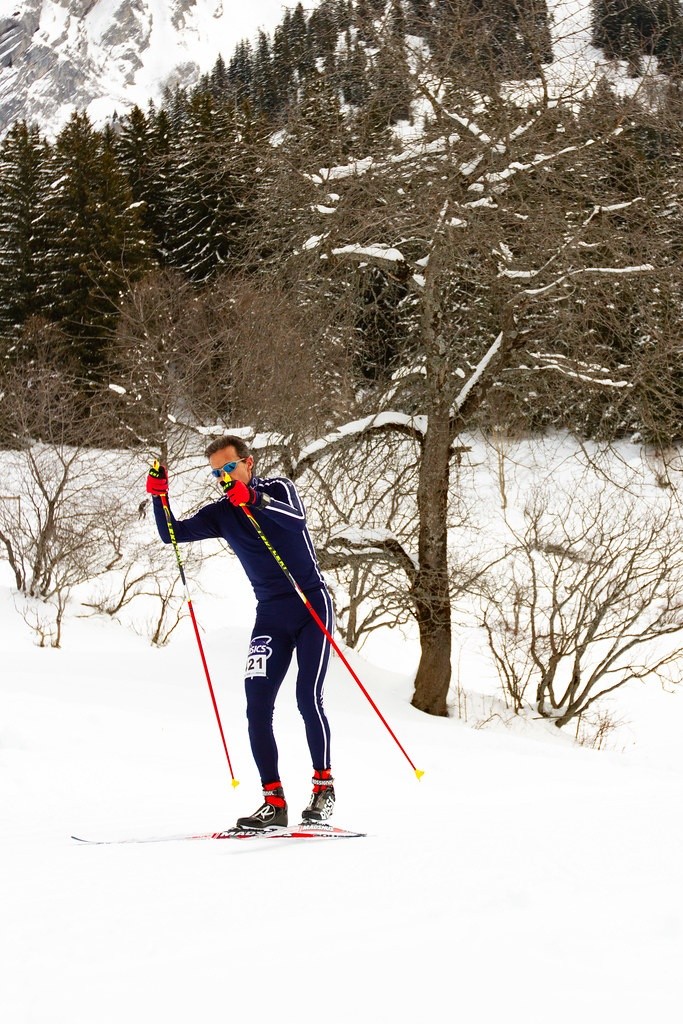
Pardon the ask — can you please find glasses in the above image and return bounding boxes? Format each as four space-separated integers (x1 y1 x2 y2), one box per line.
211 458 245 478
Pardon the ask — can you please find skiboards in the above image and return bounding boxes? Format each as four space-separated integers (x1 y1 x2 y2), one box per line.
71 826 368 844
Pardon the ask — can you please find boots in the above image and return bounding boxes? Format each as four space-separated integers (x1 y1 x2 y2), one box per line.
301 768 336 823
236 781 288 831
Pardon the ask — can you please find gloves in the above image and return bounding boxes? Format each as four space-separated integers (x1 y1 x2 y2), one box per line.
145 465 169 496
223 480 257 507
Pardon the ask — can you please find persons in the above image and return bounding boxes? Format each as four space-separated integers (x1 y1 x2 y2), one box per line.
147 435 335 832
